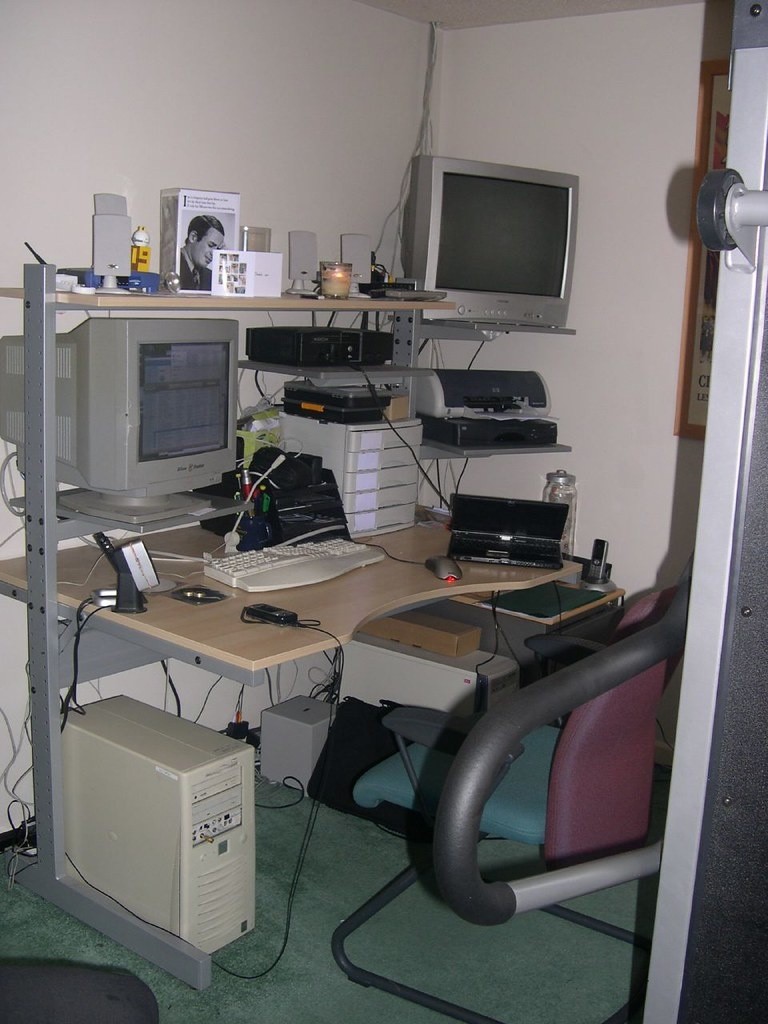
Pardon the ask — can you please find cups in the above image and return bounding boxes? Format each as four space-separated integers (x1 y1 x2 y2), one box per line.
320 260 352 300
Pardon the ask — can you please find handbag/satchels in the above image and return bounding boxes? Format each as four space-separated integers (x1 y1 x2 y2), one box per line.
307 697 437 840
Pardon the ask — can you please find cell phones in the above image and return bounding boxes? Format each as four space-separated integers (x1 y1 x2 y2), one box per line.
589 538 609 579
246 603 298 623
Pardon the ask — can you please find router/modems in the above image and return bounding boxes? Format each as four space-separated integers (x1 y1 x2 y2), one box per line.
24 242 160 292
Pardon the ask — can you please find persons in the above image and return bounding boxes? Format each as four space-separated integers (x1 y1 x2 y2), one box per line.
179 215 226 291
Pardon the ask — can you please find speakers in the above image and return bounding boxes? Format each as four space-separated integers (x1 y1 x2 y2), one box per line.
262 694 336 792
339 234 372 299
91 193 131 294
287 231 319 296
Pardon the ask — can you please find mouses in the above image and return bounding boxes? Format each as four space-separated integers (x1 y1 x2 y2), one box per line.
425 556 462 581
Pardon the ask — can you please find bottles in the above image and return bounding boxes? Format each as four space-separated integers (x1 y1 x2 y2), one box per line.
543 470 578 564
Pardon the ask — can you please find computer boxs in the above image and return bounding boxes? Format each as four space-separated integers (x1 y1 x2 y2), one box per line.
58 694 257 954
338 632 520 717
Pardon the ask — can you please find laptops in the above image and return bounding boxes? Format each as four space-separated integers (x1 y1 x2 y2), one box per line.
447 493 569 570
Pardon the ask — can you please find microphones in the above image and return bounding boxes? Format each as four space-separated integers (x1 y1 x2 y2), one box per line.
231 454 286 534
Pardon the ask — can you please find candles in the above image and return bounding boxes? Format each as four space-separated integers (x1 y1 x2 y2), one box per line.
322 267 350 294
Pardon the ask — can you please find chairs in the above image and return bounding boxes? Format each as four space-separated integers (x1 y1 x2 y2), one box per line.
330 575 692 1024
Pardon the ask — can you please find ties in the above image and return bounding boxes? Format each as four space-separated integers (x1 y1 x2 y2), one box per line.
193 268 199 288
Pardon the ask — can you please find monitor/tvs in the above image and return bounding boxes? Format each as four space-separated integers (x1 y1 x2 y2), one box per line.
0 317 239 523
399 154 580 327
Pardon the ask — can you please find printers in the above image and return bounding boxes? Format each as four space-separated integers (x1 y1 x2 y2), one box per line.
416 370 559 422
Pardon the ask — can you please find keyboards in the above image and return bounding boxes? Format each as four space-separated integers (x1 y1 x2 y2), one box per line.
204 538 385 592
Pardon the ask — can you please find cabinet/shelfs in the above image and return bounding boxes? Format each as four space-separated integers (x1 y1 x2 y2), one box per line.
0 264 595 987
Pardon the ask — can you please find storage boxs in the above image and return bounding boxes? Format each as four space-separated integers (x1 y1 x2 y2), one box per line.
280 412 424 540
161 188 242 293
385 394 410 420
358 612 482 657
